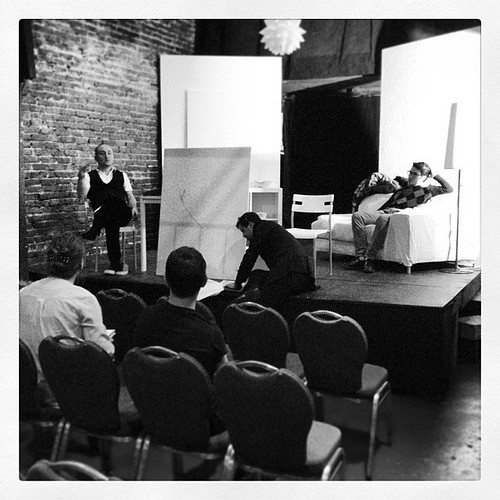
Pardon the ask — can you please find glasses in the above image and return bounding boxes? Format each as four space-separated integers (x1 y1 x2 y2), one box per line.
408 171 424 176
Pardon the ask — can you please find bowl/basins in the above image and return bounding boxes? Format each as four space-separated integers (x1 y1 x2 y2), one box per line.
254 178 272 188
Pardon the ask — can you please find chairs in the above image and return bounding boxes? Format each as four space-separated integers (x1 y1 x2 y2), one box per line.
95 289 146 365
38 336 143 481
222 301 307 386
122 345 230 481
19 339 63 479
292 310 394 480
284 194 333 281
212 360 346 481
81 201 138 271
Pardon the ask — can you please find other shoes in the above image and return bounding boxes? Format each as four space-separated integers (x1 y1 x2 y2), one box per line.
364 258 375 273
81 231 97 241
343 257 365 270
105 262 124 274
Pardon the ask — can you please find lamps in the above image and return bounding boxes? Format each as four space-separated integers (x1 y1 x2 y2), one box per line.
260 20 306 56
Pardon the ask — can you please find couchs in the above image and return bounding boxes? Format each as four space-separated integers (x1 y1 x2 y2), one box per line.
313 167 461 271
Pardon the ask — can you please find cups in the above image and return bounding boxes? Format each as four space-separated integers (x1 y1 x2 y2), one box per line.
257 212 266 219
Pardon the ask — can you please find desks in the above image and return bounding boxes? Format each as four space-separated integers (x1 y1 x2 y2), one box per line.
138 189 162 272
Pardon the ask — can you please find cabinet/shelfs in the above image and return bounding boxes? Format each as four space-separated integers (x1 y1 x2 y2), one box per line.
246 189 283 250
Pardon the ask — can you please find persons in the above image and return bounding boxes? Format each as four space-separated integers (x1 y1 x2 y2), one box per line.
19 234 114 408
19 279 33 286
77 143 138 274
121 247 230 437
224 212 315 306
343 162 452 274
